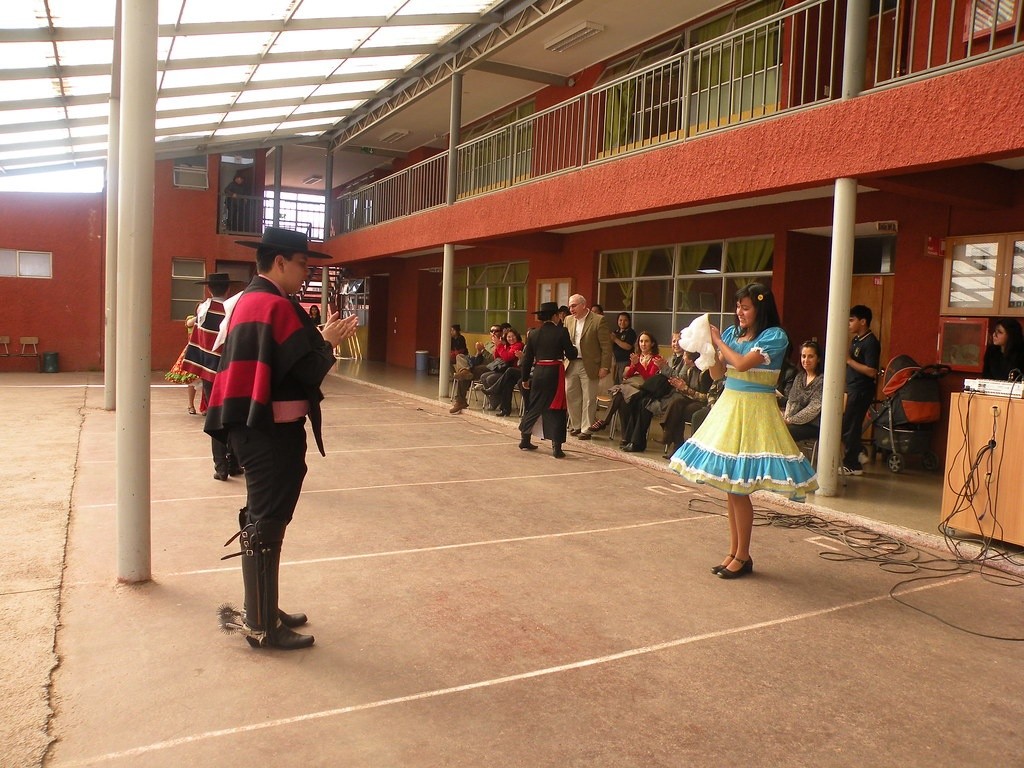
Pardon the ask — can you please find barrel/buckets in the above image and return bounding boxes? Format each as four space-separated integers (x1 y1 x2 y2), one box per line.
415 350 429 371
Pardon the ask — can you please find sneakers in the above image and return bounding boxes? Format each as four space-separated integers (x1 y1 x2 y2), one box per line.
838 465 864 477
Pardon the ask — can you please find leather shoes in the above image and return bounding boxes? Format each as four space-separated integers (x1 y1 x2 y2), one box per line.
247 623 314 649
278 609 307 626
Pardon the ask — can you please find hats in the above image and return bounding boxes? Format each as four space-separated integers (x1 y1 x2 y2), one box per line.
532 302 564 314
451 325 461 331
235 227 334 259
194 273 243 285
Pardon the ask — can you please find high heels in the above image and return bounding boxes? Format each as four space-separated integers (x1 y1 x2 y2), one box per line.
711 554 735 574
717 556 753 577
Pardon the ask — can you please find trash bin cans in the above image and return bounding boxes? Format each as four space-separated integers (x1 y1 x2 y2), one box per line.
415 351 429 371
44 352 58 373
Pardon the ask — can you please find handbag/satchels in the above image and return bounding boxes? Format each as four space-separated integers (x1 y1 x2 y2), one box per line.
486 357 513 372
640 374 672 397
622 376 645 389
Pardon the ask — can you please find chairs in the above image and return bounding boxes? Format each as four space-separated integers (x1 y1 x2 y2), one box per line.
451 373 848 488
0 336 41 373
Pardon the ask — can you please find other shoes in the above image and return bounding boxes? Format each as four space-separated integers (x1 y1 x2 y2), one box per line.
454 370 473 380
570 429 581 436
229 468 244 476
496 409 511 416
578 433 592 440
481 387 495 401
188 406 196 414
589 420 607 431
623 442 643 452
450 401 468 413
215 471 228 481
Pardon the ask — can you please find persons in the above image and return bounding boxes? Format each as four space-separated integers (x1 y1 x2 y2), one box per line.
590 304 603 316
775 341 799 406
165 274 244 416
563 294 613 440
588 331 727 460
203 227 358 649
668 284 820 579
518 302 578 459
588 330 667 436
611 312 638 386
308 304 322 328
558 305 572 323
838 305 881 475
783 340 826 442
448 323 536 417
982 317 1024 383
224 173 247 233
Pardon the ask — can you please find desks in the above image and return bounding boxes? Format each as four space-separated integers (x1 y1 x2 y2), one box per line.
941 392 1024 551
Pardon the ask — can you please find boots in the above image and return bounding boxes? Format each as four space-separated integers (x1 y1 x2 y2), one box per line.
553 440 565 457
519 433 538 450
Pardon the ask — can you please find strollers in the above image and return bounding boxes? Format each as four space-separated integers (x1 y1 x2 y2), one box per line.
860 354 952 474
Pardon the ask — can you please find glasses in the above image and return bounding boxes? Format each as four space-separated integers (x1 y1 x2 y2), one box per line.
490 330 501 333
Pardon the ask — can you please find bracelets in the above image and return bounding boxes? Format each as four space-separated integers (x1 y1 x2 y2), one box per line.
602 367 610 373
